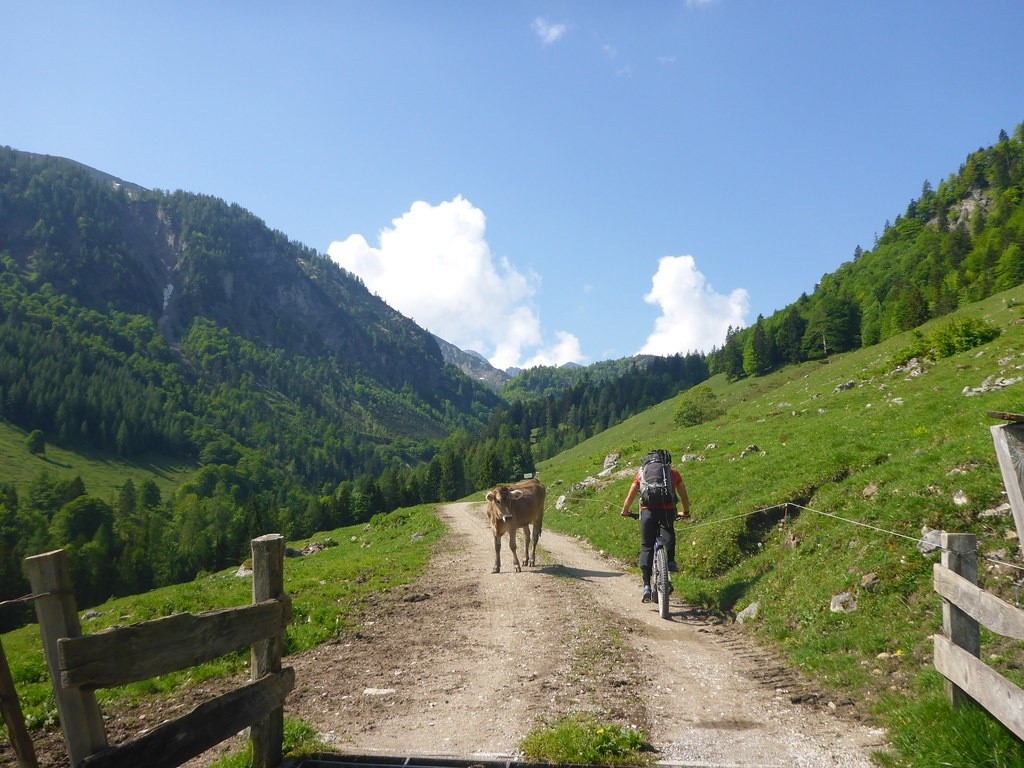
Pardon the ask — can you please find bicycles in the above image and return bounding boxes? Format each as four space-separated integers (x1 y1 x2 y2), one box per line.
620 511 692 618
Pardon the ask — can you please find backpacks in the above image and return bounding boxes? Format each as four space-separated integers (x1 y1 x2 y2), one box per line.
639 450 679 508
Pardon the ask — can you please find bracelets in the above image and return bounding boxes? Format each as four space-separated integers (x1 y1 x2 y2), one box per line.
684 511 691 515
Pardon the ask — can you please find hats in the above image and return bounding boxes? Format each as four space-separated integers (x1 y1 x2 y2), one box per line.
658 449 671 464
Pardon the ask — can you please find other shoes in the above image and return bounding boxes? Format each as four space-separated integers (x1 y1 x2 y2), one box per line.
668 561 679 572
642 585 652 603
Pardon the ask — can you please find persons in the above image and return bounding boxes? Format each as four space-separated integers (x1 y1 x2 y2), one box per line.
620 448 691 603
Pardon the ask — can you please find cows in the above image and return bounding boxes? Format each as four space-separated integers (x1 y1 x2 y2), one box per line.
485 474 546 575
